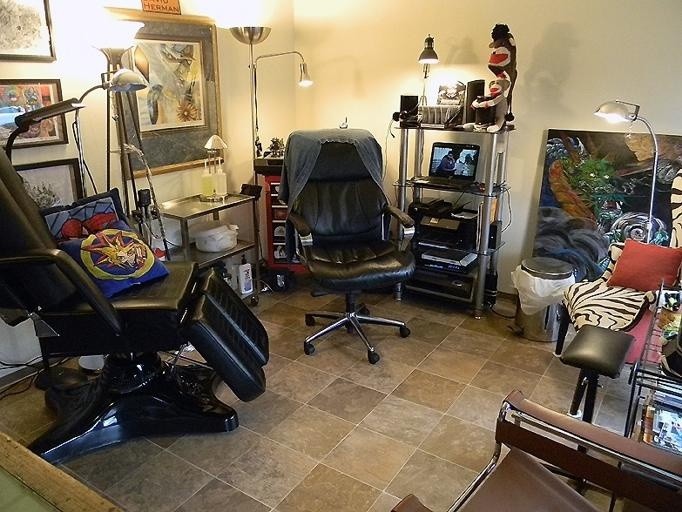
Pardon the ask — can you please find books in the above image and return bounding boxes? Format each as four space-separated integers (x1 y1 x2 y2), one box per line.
634 376 681 456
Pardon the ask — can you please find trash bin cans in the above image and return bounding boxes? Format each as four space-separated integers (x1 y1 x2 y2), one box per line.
511 256 575 342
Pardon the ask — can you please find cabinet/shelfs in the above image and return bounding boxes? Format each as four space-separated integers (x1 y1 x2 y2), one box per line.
262 174 308 289
395 125 518 320
146 192 268 310
385 387 682 512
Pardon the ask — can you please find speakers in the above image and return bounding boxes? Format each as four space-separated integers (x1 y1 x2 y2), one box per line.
401 96 418 119
475 96 495 125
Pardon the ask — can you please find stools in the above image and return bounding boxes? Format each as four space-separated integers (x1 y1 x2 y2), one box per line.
560 324 637 454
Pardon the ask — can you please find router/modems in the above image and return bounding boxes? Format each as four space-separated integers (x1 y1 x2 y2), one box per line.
488 221 502 249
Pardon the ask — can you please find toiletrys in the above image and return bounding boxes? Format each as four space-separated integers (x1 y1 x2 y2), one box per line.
223 256 238 291
237 253 254 295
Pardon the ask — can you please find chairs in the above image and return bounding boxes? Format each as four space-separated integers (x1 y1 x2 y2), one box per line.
553 168 682 369
283 126 420 365
1 142 271 465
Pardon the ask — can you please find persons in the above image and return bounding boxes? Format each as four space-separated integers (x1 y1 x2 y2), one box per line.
438 150 455 175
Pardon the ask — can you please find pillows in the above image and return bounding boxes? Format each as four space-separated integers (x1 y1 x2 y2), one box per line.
57 221 171 300
608 236 680 293
40 188 132 244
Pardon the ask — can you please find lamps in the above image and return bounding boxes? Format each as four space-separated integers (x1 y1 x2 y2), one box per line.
72 66 148 203
415 33 440 117
595 99 658 245
230 26 313 270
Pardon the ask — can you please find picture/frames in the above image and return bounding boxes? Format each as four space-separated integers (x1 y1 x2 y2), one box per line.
0 1 58 67
12 157 85 213
104 4 226 180
0 78 80 150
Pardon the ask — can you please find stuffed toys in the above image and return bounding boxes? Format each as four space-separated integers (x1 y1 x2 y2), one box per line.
487 24 518 121
463 77 510 134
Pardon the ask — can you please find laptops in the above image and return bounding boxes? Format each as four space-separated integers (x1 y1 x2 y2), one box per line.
412 142 480 190
